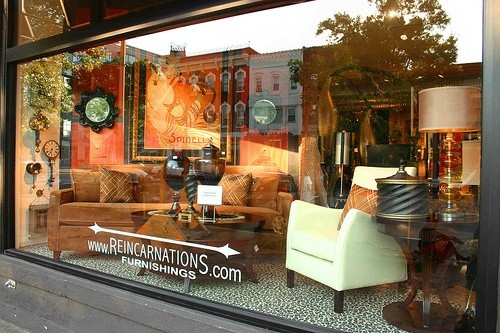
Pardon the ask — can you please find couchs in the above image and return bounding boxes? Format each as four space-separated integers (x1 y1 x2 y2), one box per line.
47 164 293 261
284 166 417 313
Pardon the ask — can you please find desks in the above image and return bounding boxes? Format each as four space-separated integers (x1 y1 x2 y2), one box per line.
372 216 479 333
130 211 265 293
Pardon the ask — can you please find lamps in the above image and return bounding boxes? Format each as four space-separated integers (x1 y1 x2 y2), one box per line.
419 87 482 218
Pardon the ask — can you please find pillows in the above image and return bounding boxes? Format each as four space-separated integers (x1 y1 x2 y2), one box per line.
248 175 281 211
70 168 99 202
99 164 134 203
217 173 252 205
337 183 377 232
